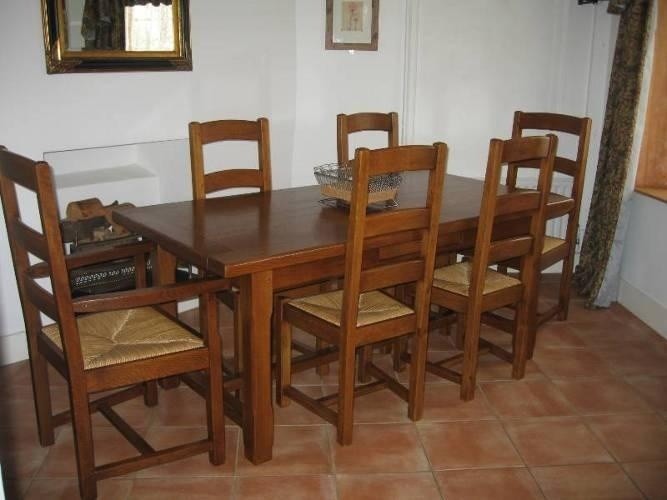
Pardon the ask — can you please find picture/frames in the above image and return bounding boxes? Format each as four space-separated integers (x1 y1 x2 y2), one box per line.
323 0 383 54
39 0 197 75
